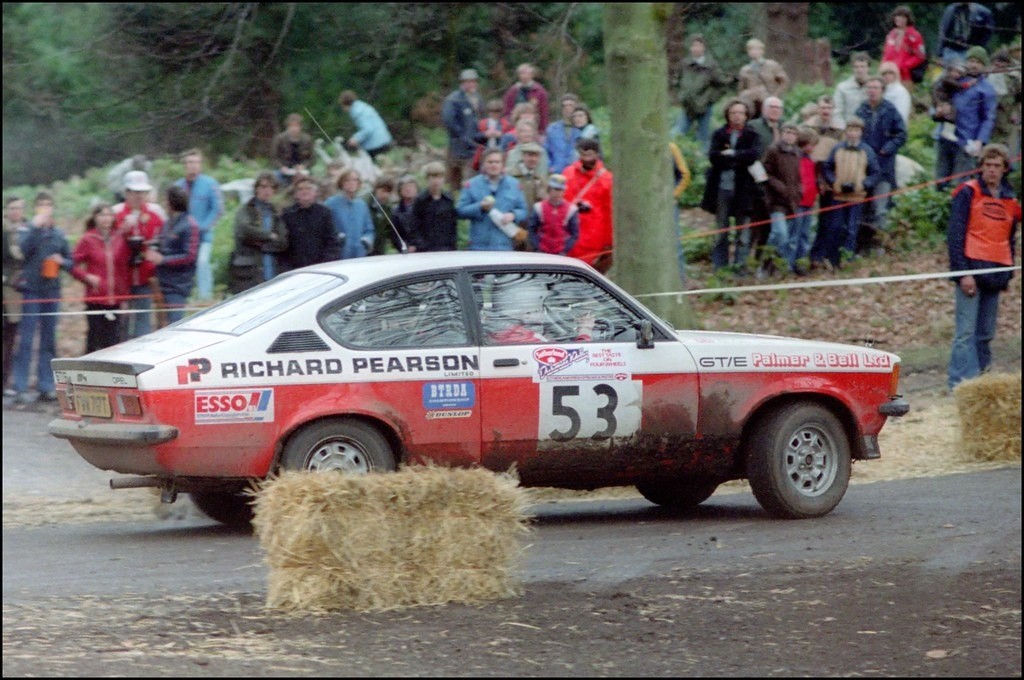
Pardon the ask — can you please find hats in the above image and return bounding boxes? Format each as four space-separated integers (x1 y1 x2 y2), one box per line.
457 70 480 82
548 174 568 190
965 46 989 65
122 170 152 193
521 143 545 153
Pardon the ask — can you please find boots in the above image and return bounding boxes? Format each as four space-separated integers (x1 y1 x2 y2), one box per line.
941 122 959 142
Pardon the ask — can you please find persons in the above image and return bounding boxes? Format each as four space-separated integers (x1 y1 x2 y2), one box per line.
943 142 1023 392
928 46 1000 192
669 33 728 142
323 63 613 277
266 111 318 188
706 61 912 280
936 3 994 53
482 276 595 343
879 5 926 94
829 50 873 131
3 148 339 406
669 141 691 281
336 90 395 163
737 37 791 98
930 58 979 141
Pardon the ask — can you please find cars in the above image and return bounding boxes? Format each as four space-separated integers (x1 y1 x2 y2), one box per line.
47 250 911 531
